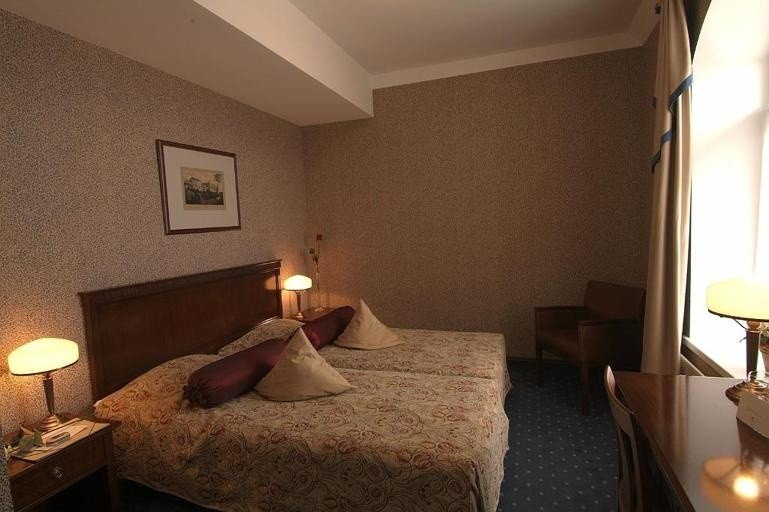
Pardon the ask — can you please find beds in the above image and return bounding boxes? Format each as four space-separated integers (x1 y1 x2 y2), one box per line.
75 257 512 512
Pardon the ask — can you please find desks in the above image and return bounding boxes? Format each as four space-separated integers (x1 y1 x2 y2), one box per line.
611 368 768 512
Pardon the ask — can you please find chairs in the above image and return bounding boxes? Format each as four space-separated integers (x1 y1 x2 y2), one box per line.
594 363 649 511
530 276 650 419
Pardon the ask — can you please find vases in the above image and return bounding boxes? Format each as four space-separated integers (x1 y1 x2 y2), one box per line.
312 271 324 313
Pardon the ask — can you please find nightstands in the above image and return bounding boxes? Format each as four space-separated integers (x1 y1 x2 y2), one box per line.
289 305 335 324
0 408 127 512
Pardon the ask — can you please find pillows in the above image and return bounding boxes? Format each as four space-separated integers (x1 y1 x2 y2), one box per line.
251 326 358 403
288 304 358 350
183 333 287 411
330 296 406 352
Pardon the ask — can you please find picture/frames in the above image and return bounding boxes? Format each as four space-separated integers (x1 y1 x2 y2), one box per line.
152 132 243 239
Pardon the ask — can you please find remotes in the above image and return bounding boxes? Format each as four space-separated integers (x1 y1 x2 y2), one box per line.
46 431 70 446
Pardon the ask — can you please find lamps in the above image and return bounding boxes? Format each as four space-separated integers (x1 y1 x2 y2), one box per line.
703 270 769 402
280 270 312 320
697 443 768 512
6 333 86 432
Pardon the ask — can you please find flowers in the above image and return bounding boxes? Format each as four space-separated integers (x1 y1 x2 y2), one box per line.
306 229 329 293
